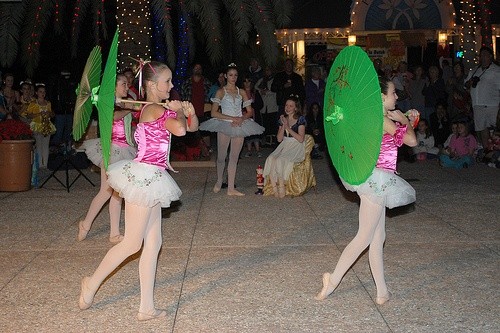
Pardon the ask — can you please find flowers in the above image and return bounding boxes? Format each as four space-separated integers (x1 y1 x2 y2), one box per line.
0 120 36 145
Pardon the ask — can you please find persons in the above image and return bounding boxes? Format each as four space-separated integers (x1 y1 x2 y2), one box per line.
240 57 326 150
263 97 307 199
178 62 213 160
20 83 56 169
0 75 20 119
80 61 199 320
373 46 500 169
208 72 226 102
78 72 142 243
317 77 419 305
122 67 142 101
18 79 36 119
199 62 265 197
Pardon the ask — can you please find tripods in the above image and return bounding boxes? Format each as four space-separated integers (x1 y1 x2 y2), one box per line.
40 110 95 193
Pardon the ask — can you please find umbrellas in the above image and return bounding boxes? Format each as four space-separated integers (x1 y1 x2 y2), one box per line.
72 45 103 141
91 28 168 170
323 45 407 186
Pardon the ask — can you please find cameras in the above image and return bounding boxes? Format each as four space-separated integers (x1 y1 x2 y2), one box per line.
471 76 480 88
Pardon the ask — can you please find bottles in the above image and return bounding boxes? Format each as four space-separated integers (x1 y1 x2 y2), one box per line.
256 164 264 186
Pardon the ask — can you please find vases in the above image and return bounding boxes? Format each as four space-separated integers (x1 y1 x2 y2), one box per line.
0 139 36 192
